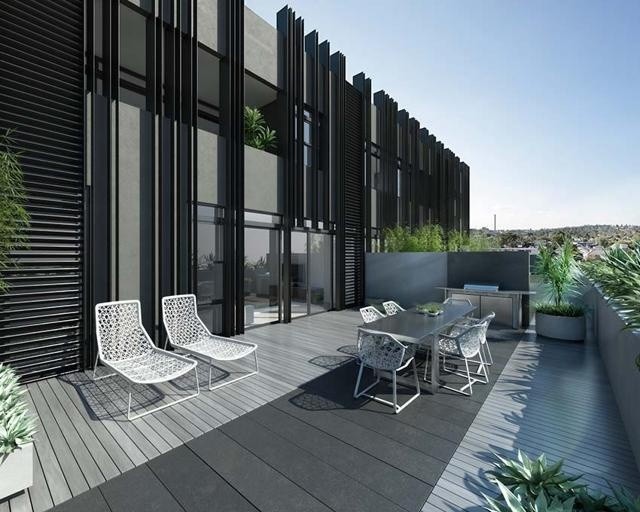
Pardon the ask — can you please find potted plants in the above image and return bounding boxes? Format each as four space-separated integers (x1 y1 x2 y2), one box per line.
0 361 40 501
527 230 595 342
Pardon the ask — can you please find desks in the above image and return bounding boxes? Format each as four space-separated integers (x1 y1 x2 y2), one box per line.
435 286 536 329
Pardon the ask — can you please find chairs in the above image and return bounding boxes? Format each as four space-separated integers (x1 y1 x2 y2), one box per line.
353 298 495 415
162 294 259 391
93 300 200 421
